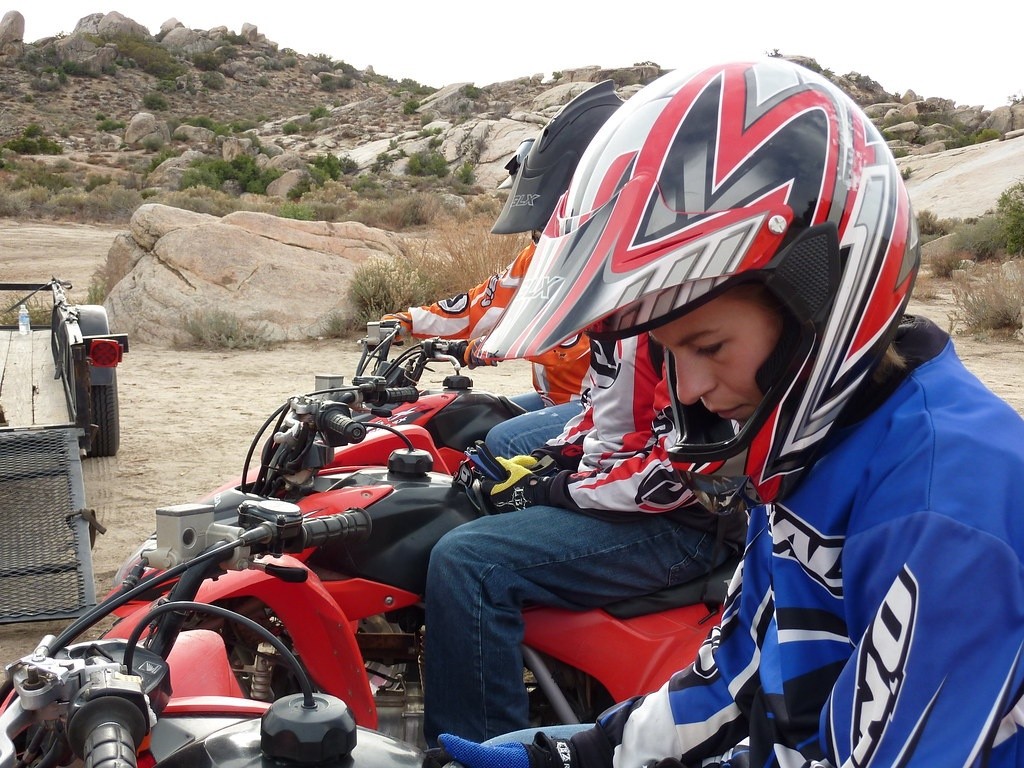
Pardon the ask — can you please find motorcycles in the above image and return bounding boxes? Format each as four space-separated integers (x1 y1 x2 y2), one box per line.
0 313 728 768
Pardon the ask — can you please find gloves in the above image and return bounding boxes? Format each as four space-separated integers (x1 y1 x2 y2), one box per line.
438 731 579 768
481 455 574 516
381 312 413 346
446 341 467 367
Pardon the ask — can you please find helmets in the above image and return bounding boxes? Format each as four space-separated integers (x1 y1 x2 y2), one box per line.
469 54 920 517
490 79 627 246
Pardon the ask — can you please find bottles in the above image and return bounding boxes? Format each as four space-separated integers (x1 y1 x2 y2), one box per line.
18 305 30 335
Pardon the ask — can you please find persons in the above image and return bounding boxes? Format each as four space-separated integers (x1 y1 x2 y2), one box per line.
379 55 1024 768
